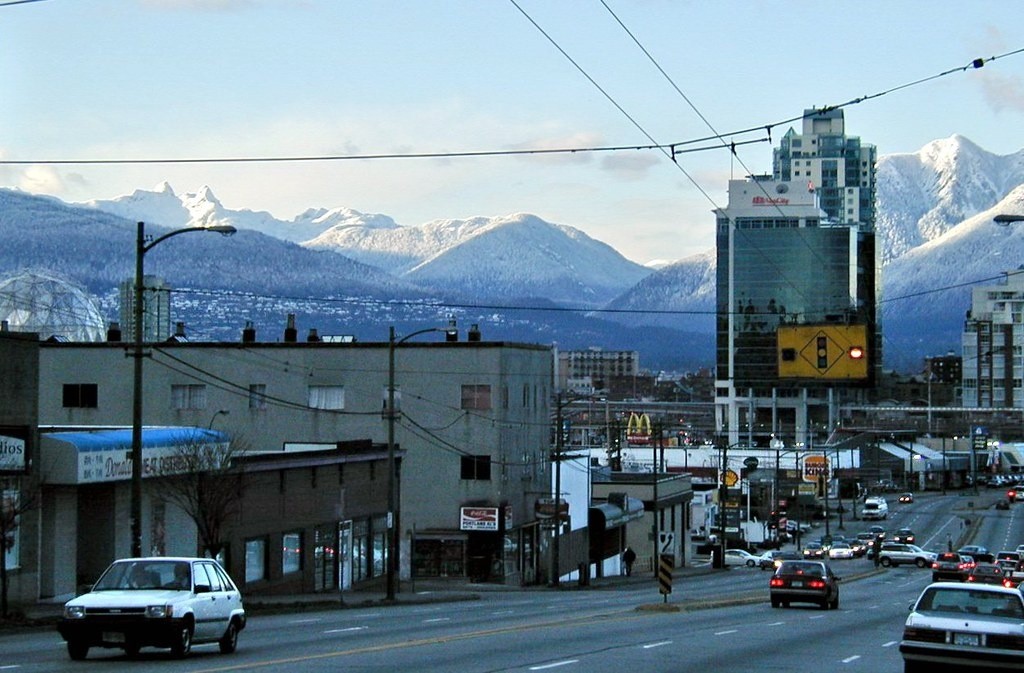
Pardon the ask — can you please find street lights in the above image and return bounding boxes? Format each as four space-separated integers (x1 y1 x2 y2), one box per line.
128 220 240 558
774 438 887 550
651 420 694 579
720 439 759 569
385 322 457 600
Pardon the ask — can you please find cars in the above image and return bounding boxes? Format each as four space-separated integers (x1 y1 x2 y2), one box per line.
779 519 1024 606
53 555 249 662
770 558 843 610
899 493 914 503
996 499 1010 511
898 582 1024 673
710 548 763 568
976 473 1024 502
759 549 806 573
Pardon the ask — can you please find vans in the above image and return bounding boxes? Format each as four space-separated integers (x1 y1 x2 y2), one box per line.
861 495 889 520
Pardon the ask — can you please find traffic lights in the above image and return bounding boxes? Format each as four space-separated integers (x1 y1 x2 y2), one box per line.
782 348 794 360
768 520 778 529
850 346 863 359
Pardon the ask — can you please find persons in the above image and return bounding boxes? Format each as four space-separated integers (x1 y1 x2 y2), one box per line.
871 533 884 570
946 533 954 552
791 527 797 544
622 547 636 577
